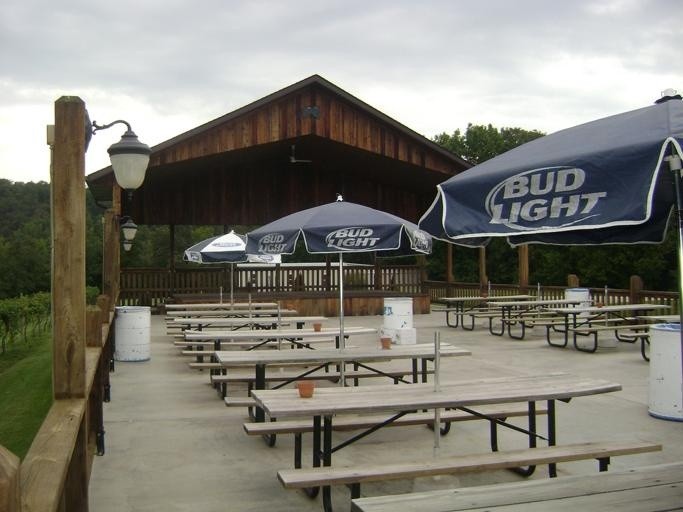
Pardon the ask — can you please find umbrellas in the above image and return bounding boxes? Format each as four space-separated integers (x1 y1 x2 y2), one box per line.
417 86 683 385
242 191 432 387
183 228 274 311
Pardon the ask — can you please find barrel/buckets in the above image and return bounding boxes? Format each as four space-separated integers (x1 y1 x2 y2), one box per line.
647 323 683 422
113 305 152 362
563 287 591 323
381 297 414 344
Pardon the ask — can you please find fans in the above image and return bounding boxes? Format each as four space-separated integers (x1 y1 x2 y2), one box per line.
278 141 312 164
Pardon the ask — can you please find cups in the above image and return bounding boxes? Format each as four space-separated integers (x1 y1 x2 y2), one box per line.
596 303 602 308
298 382 315 398
313 323 321 332
483 293 487 298
380 337 392 350
287 304 293 311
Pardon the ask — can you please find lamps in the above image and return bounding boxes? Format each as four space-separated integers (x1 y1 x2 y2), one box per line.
120 239 136 252
90 118 152 200
117 214 138 242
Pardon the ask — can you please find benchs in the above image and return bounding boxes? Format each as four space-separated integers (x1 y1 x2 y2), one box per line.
529 315 647 347
244 400 548 496
471 310 559 336
225 395 256 408
164 315 272 322
502 314 606 339
432 305 522 326
277 439 663 511
455 309 539 330
620 332 651 362
179 350 219 356
567 323 652 352
174 338 335 348
167 320 290 349
187 358 394 398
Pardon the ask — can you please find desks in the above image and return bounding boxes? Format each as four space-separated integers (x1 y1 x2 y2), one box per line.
185 326 378 386
174 316 328 363
439 294 537 331
214 342 472 446
489 299 594 340
351 461 683 512
636 315 680 325
250 373 621 511
541 304 671 352
164 303 277 330
166 309 297 335
